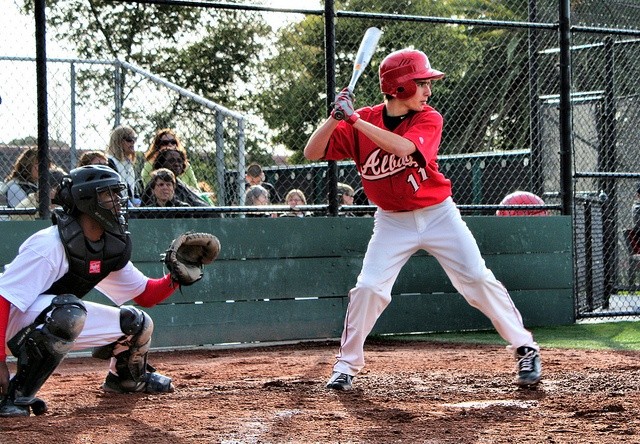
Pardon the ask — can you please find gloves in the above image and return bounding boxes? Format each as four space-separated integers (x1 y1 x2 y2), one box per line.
331 88 356 121
336 95 360 126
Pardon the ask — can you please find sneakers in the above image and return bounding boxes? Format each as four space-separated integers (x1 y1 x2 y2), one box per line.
103 372 176 394
2 395 47 418
512 346 543 385
325 371 354 392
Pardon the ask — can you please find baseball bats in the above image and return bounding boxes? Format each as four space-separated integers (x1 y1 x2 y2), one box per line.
333 28 380 121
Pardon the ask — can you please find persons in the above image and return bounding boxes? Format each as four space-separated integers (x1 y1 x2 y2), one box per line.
337 182 356 218
278 189 311 218
140 128 215 207
4 145 38 208
77 151 108 167
8 166 68 221
144 167 192 207
303 47 544 394
140 147 221 219
244 185 278 218
106 125 142 208
0 164 222 418
244 163 281 205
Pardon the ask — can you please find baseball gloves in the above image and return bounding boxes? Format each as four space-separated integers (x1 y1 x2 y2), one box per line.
166 233 221 285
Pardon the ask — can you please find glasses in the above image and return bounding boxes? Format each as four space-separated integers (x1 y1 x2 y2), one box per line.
123 136 138 143
166 158 183 164
159 139 177 146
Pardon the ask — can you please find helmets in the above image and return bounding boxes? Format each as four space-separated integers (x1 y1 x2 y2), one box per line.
51 164 133 240
379 48 446 101
